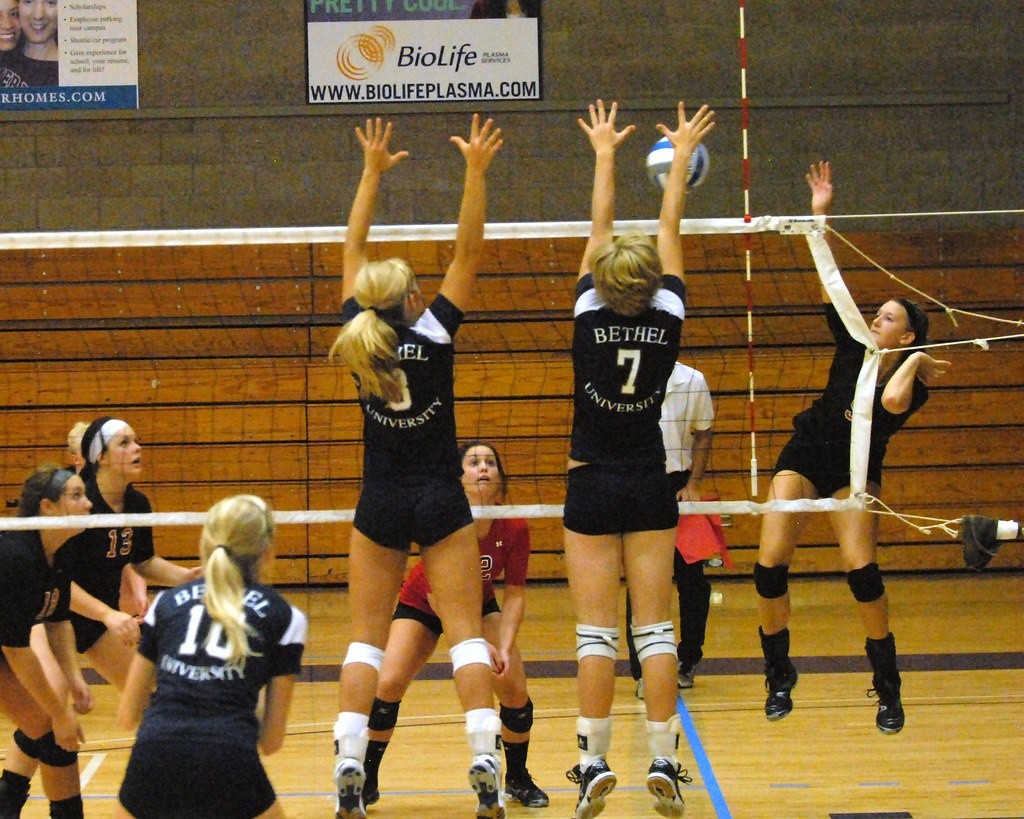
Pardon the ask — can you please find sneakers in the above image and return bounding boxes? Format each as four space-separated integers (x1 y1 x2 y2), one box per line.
635 677 645 699
333 757 367 819
645 757 692 819
504 768 549 808
565 760 617 819
469 753 506 819
676 657 699 689
867 677 904 735
361 781 380 811
763 664 800 720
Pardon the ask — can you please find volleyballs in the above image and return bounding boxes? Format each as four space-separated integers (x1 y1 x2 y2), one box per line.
646 136 709 191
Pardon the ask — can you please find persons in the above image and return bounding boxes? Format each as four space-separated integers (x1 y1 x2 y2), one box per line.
626 361 715 699
117 495 309 819
753 159 951 735
330 113 506 819
362 441 550 807
0 0 59 87
562 100 717 819
61 416 204 693
0 467 94 819
